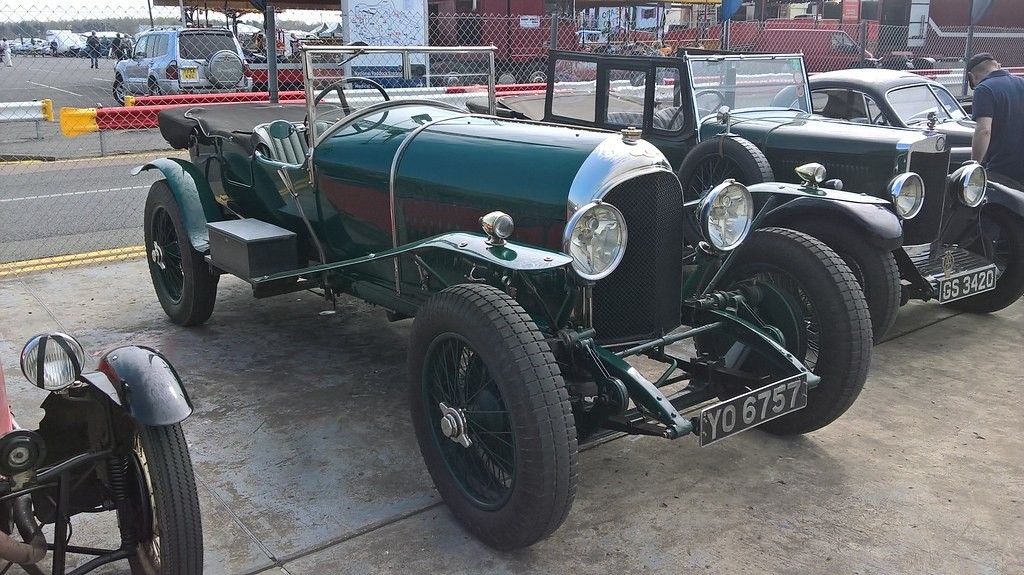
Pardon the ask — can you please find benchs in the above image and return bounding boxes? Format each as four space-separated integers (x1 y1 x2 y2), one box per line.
253 121 326 164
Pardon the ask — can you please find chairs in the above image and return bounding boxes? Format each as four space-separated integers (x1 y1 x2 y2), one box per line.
656 106 710 129
607 111 664 129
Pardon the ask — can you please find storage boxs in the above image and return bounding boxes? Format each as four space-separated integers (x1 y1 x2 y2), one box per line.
205 218 299 281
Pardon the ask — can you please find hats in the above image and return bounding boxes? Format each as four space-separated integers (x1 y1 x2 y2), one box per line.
967 53 995 90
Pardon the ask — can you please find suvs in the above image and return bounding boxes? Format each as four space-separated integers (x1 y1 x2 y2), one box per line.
113 25 253 106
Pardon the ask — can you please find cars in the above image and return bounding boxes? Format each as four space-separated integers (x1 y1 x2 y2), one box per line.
466 47 1024 347
770 68 987 173
130 40 872 552
0 30 137 54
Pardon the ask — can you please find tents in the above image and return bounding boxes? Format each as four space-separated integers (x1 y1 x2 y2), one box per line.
319 22 343 39
311 23 328 37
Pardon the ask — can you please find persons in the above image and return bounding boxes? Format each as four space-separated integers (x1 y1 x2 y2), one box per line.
290 33 300 63
965 52 1024 193
2 38 12 66
111 33 133 60
86 30 101 69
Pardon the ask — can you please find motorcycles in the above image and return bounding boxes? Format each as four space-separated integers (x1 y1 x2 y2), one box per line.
0 332 205 575
